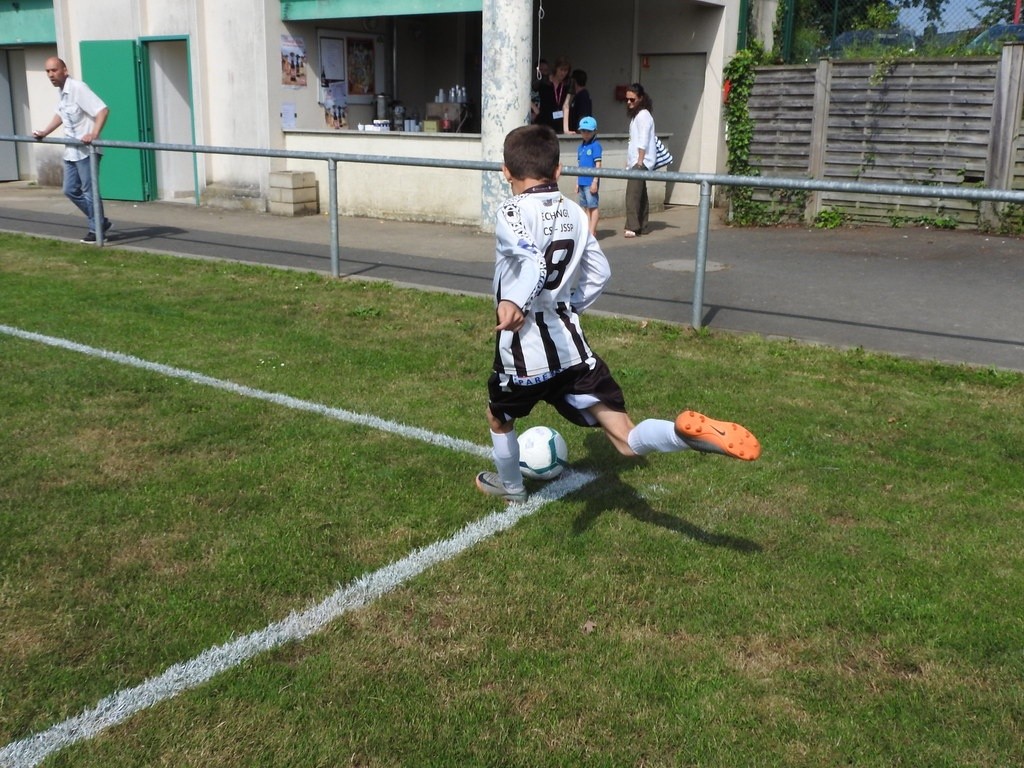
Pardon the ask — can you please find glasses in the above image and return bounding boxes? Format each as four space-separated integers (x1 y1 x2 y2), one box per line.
624 98 639 102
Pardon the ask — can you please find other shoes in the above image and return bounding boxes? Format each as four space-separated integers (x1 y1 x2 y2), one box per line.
624 230 635 238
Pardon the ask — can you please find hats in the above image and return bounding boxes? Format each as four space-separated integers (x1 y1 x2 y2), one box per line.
578 117 597 131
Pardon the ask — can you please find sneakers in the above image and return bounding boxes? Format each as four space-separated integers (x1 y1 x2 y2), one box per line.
80 232 108 243
476 470 527 504
105 222 112 231
674 411 762 461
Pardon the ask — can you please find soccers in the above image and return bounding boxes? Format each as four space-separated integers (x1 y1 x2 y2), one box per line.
517 425 567 481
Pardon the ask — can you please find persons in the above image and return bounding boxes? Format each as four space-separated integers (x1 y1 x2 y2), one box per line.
475 125 766 503
32 56 111 244
623 83 656 238
530 56 592 135
576 116 602 237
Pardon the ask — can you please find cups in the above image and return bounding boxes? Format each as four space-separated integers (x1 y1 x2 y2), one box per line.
358 125 364 131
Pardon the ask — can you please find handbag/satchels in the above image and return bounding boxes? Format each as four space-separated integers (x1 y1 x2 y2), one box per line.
653 136 674 169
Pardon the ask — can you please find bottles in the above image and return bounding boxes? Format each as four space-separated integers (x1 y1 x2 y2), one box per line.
332 105 347 129
442 112 451 132
285 53 303 81
